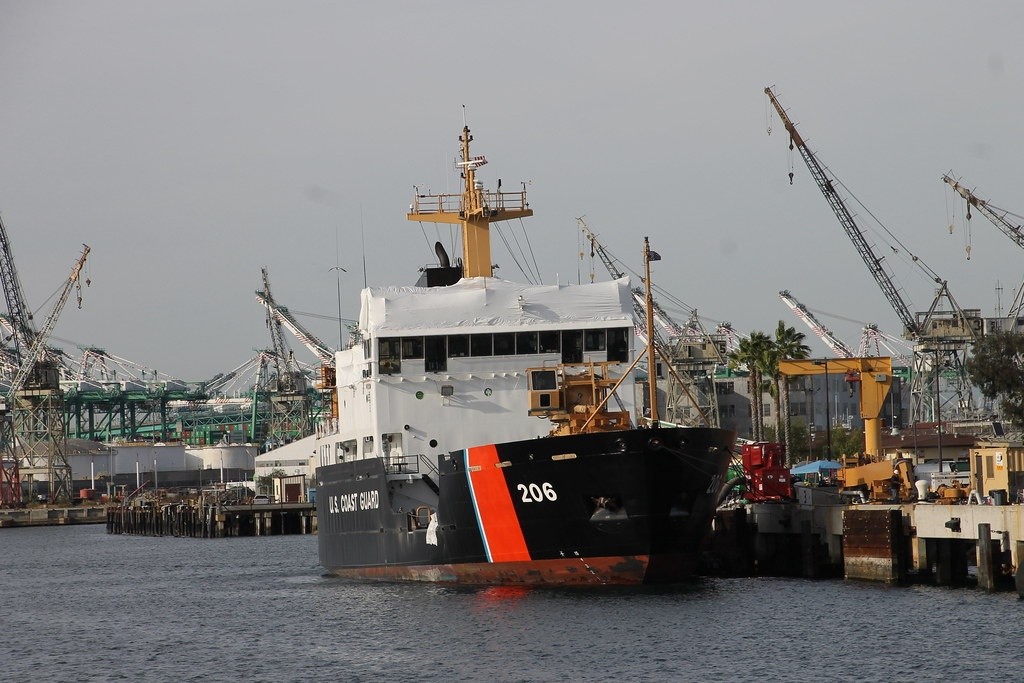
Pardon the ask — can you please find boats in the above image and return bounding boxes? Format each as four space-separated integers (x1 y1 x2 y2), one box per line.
313 106 738 588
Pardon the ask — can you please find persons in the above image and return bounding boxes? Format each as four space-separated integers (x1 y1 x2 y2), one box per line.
890 469 900 502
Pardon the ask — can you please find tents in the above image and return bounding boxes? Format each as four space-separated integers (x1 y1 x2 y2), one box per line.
790 461 843 487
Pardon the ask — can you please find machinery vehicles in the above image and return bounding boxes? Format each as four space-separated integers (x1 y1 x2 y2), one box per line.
0 218 359 510
572 84 1024 504
